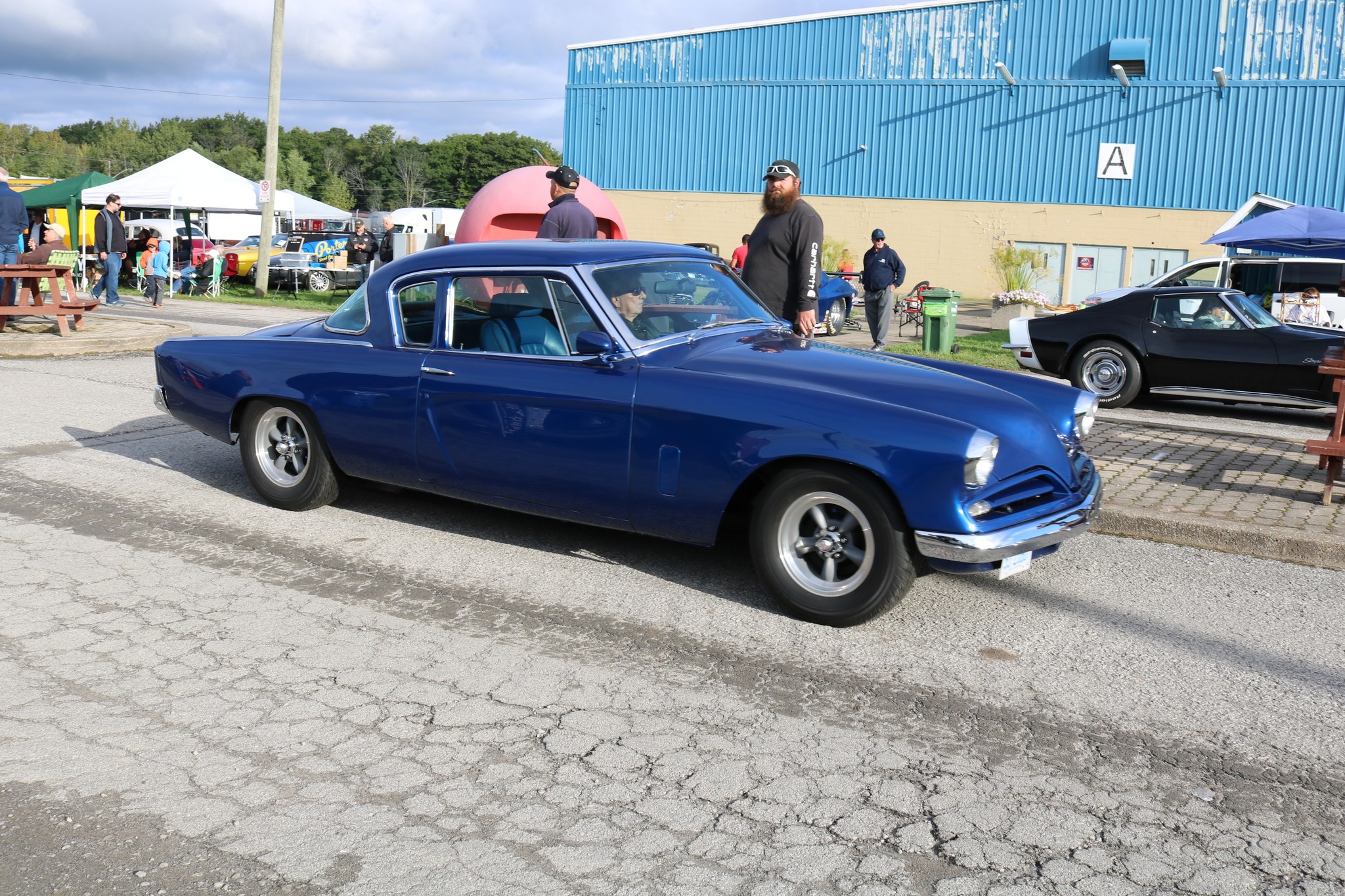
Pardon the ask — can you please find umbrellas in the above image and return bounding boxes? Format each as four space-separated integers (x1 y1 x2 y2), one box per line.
1200 206 1345 260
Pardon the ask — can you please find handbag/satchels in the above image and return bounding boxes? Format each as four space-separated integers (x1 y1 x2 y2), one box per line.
835 262 845 278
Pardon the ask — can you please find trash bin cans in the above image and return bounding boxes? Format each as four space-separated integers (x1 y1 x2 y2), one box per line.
918 288 961 354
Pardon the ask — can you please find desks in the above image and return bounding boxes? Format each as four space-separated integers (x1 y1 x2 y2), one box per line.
268 266 361 305
78 254 99 293
0 264 84 337
1316 358 1345 506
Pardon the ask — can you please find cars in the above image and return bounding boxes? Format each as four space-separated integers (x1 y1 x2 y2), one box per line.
682 242 732 288
656 270 694 305
672 272 858 333
123 218 386 292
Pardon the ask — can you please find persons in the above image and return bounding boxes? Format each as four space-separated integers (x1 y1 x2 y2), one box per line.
737 330 812 353
344 214 398 288
535 166 598 240
87 194 127 307
837 229 906 352
608 273 660 340
730 159 824 337
139 237 229 308
0 167 70 319
1190 297 1240 330
1285 287 1332 327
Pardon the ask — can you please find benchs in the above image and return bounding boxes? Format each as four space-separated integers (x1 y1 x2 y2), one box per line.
1303 440 1345 457
61 299 100 307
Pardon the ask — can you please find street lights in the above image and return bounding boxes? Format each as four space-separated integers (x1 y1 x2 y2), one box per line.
422 188 447 208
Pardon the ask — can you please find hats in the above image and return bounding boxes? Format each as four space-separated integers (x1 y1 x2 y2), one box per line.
33 210 44 216
546 165 579 189
146 244 155 248
872 228 885 239
355 219 364 228
43 223 65 237
762 158 799 181
205 249 220 258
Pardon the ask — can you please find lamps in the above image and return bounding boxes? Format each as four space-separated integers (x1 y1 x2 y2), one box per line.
1213 67 1227 98
1112 64 1130 98
995 61 1016 96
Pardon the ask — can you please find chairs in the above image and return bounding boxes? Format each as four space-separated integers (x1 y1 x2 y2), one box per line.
18 250 80 319
894 281 936 337
480 293 567 356
131 252 149 294
223 253 239 290
1166 310 1184 328
189 256 223 298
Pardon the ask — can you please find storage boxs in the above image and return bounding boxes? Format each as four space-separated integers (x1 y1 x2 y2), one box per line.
326 250 348 269
86 268 105 285
279 252 316 266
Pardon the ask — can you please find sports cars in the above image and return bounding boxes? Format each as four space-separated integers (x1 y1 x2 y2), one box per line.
999 286 1345 409
152 239 1105 627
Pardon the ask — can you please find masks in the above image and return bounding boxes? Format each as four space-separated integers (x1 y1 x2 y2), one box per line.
1304 298 1317 304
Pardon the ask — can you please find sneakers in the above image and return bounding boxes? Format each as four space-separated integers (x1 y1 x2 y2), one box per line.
870 342 885 352
105 299 125 307
88 288 98 300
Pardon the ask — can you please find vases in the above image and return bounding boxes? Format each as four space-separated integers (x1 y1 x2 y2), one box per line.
991 299 1035 330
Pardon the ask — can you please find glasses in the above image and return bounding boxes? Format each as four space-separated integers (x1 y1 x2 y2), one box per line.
874 238 884 241
112 202 120 206
1308 296 1318 299
1211 305 1222 309
766 165 797 178
616 286 646 296
34 215 43 217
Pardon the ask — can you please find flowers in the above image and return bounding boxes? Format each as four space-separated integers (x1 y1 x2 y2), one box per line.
990 289 1050 310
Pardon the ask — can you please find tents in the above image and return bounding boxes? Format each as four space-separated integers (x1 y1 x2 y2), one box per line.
81 148 299 297
17 172 119 291
280 188 353 236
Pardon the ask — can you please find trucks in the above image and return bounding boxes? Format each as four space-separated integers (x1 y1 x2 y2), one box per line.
7 178 125 269
1082 255 1345 335
380 208 466 246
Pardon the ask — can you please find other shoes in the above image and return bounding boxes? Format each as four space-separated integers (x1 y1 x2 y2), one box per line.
150 300 164 308
129 282 137 288
6 315 15 321
167 269 181 279
164 290 173 296
143 296 153 304
